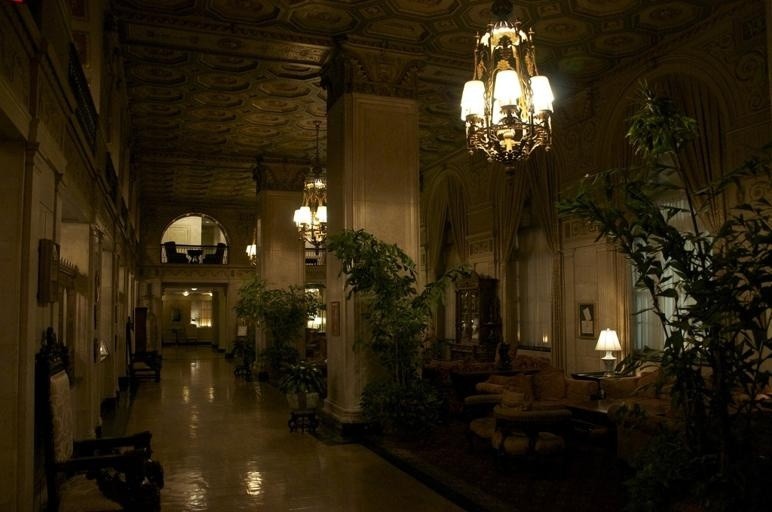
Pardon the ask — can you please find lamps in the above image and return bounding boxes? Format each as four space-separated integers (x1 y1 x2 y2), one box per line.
595 328 623 378
458 0 557 163
94 337 110 365
291 120 328 256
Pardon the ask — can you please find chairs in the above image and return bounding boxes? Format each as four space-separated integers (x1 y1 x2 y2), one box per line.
35 325 169 512
126 315 160 382
163 241 226 264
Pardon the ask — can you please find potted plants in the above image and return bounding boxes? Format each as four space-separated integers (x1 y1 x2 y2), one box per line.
325 221 470 447
231 276 328 433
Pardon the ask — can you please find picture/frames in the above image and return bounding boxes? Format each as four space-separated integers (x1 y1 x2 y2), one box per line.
577 301 597 339
330 302 340 336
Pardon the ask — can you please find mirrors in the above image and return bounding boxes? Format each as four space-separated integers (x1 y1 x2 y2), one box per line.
455 270 484 344
